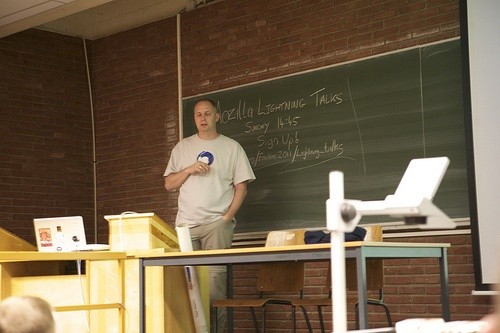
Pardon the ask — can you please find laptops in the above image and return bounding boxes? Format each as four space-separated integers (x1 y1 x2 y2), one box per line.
33 216 110 252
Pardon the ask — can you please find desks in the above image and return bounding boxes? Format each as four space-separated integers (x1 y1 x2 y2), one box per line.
0 250 127 333
134 240 453 333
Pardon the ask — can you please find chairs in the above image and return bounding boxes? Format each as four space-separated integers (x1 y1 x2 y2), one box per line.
208 228 314 333
291 225 394 333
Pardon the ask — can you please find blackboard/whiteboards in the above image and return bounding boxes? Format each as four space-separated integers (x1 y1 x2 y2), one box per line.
182 36 472 245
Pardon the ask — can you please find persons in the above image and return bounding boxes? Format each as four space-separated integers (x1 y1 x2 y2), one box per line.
162 99 256 333
0 295 56 333
